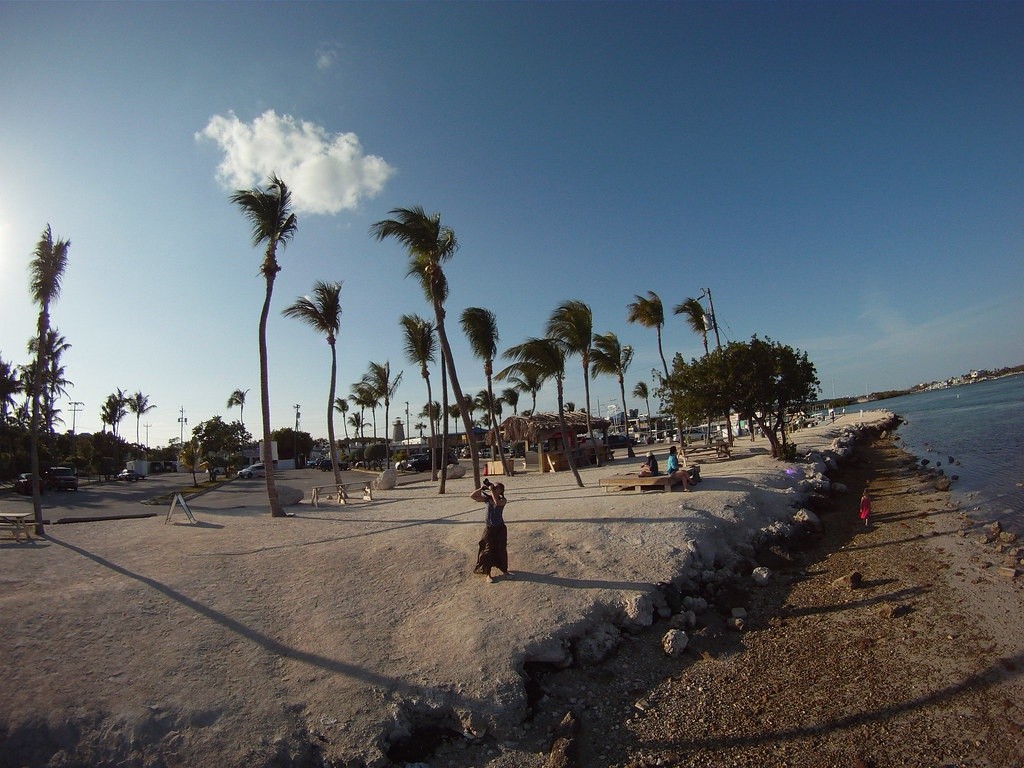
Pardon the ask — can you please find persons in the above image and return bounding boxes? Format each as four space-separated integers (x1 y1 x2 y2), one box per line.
471 482 514 583
668 445 697 492
859 488 870 528
641 451 658 475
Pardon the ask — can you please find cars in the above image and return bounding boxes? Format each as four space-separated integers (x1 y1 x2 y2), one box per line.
238 463 265 479
672 429 706 443
462 448 491 458
47 467 78 493
601 435 638 448
395 449 459 473
319 459 348 472
510 442 526 458
15 473 45 495
118 469 139 481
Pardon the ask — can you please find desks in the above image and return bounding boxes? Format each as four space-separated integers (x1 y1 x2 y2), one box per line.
0 513 34 543
487 460 514 475
679 442 732 465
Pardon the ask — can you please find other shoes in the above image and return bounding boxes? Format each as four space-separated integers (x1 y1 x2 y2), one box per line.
683 489 691 492
503 573 514 580
689 482 697 486
486 576 493 583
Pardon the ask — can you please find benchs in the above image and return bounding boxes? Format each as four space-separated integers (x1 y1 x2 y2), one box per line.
310 480 372 508
0 523 42 526
680 447 719 455
598 465 701 493
0 526 29 530
684 449 733 458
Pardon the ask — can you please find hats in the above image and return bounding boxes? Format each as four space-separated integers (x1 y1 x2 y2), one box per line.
646 451 653 457
864 488 870 493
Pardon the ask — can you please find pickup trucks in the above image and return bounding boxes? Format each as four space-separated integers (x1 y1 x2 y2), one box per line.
307 458 326 470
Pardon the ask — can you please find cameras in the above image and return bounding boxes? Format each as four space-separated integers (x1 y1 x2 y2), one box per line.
483 478 494 489
